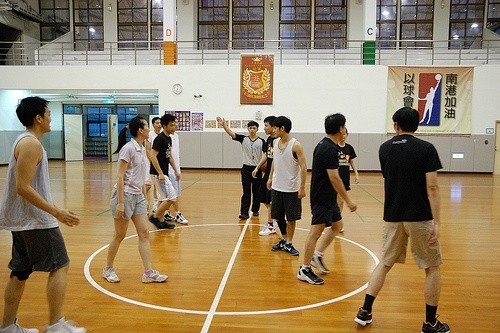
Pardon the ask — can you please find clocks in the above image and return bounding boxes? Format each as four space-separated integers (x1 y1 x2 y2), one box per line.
172 84 183 95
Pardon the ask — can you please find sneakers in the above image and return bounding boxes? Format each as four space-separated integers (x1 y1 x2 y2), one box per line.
271 238 286 250
142 270 168 282
164 213 173 220
44 316 87 333
103 266 120 283
354 306 373 326
259 223 276 236
148 214 164 229
284 242 299 256
311 254 327 275
152 204 158 212
297 266 324 285
239 213 249 218
253 212 258 215
421 314 450 333
273 219 278 228
174 210 188 224
162 221 175 228
0 318 39 333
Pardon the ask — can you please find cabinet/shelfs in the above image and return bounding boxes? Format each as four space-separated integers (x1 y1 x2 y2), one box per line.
85 140 109 157
175 131 496 173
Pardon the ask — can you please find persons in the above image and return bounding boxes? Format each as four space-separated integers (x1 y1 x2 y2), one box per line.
337 127 360 233
0 96 87 333
267 116 307 256
296 113 357 285
252 116 278 235
102 115 168 283
215 116 267 220
354 106 451 333
148 114 189 229
